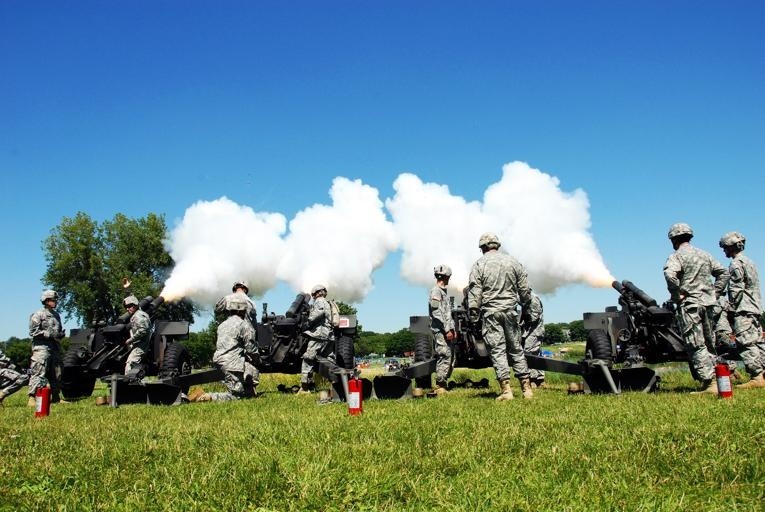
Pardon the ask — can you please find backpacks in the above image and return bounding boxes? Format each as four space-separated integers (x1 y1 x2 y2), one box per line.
326 299 340 328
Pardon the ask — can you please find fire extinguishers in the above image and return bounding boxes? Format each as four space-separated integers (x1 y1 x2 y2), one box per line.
714 353 733 399
348 369 362 417
35 376 51 417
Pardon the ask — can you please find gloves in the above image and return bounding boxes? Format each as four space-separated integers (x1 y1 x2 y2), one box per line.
470 323 481 340
518 312 534 331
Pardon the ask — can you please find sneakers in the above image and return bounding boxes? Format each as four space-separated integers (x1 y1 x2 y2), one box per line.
57 399 71 404
736 373 764 390
531 381 547 389
28 396 36 406
689 380 718 395
433 385 449 393
296 387 311 395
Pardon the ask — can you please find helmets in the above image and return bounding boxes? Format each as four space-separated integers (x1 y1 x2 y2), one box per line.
668 223 694 238
479 232 500 247
229 298 248 311
123 296 139 306
434 264 452 277
718 232 744 248
40 290 58 302
232 280 249 295
311 285 327 295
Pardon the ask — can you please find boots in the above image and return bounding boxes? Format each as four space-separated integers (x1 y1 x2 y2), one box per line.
520 377 533 399
0 390 7 410
188 388 210 402
495 380 514 400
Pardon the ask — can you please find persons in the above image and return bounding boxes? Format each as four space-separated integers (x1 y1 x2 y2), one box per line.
469 232 535 401
428 264 458 396
215 281 260 342
518 286 547 392
297 287 340 395
124 294 153 377
185 300 260 402
26 290 70 408
664 222 765 394
0 350 29 403
